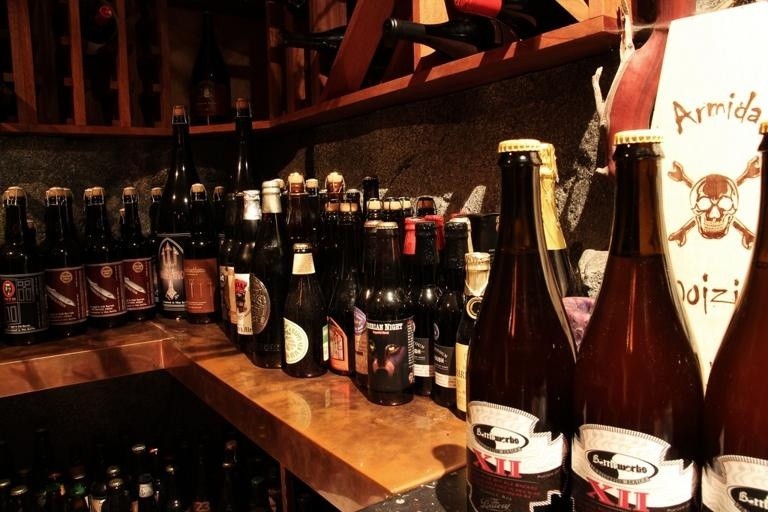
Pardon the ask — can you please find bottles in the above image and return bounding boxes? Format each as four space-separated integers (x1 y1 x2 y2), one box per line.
575 126 699 504
2 437 263 510
537 141 577 301
384 15 500 56
183 173 491 416
466 143 576 512
221 96 273 199
158 107 201 320
701 129 765 507
0 184 158 344
280 24 344 58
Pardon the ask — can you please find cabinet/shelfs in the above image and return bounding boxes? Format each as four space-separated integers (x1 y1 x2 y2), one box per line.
1 2 645 144
